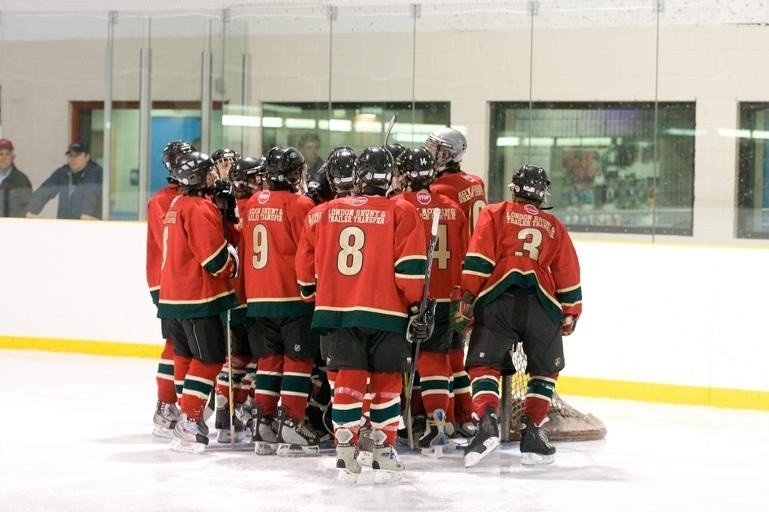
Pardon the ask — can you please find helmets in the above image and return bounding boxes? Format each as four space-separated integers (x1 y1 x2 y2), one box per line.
506 164 552 204
163 140 437 198
421 128 467 175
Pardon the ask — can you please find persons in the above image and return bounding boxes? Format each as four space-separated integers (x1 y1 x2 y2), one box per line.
25 142 102 222
147 124 584 477
1 138 33 218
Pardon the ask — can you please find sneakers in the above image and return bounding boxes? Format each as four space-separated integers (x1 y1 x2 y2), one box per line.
215 400 478 446
519 413 555 456
173 412 209 445
462 406 498 455
153 400 180 429
335 444 362 474
371 444 405 471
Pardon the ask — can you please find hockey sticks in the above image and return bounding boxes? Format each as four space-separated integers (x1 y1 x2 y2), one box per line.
397 207 441 431
384 114 400 167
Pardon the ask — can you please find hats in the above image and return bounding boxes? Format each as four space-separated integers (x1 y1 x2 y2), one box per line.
65 141 90 156
0 139 13 149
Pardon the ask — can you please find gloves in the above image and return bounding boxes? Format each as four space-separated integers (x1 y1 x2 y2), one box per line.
560 314 576 336
408 299 437 342
448 285 475 333
